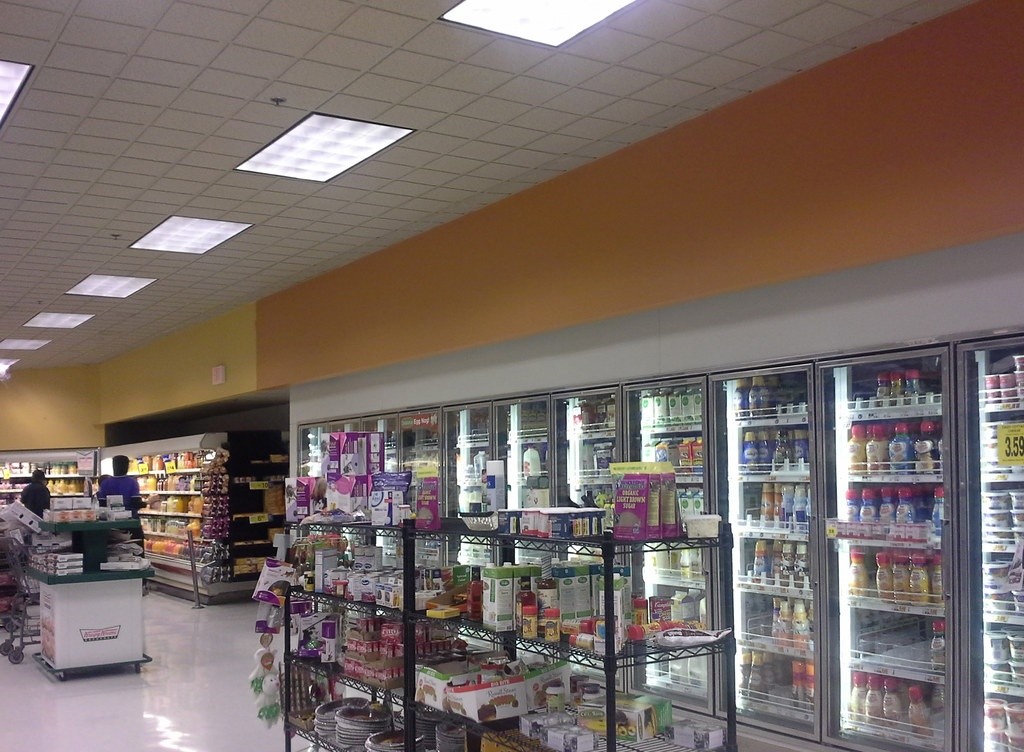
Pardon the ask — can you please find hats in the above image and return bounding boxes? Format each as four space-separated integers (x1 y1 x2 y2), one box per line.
31 470 45 482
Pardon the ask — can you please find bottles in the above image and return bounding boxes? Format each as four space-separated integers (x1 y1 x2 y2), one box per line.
516 576 536 635
523 606 538 639
545 608 560 642
467 567 482 622
736 366 945 748
581 683 601 703
537 555 557 636
546 683 565 712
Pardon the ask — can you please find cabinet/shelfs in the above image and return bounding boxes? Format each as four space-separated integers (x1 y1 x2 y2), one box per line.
97 432 290 606
284 336 1024 752
26 517 154 584
0 445 96 511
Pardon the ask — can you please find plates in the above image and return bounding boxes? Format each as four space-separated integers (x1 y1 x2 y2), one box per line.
314 698 467 752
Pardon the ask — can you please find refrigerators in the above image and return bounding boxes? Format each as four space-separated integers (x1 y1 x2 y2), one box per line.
290 236 1024 752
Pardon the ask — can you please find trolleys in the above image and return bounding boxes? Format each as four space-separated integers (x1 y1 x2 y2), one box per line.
0 534 150 664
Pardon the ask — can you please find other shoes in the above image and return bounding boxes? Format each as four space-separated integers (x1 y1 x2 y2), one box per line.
142 584 149 596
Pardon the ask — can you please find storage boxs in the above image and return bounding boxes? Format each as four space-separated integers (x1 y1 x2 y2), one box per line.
348 626 383 642
315 546 338 594
344 647 380 663
371 489 404 526
446 675 529 724
284 477 318 525
415 658 481 713
362 673 405 690
355 547 382 572
578 692 672 742
522 656 571 714
326 430 385 514
44 494 134 521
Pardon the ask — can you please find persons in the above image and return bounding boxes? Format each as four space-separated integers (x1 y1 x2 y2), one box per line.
93 455 162 597
21 470 50 519
